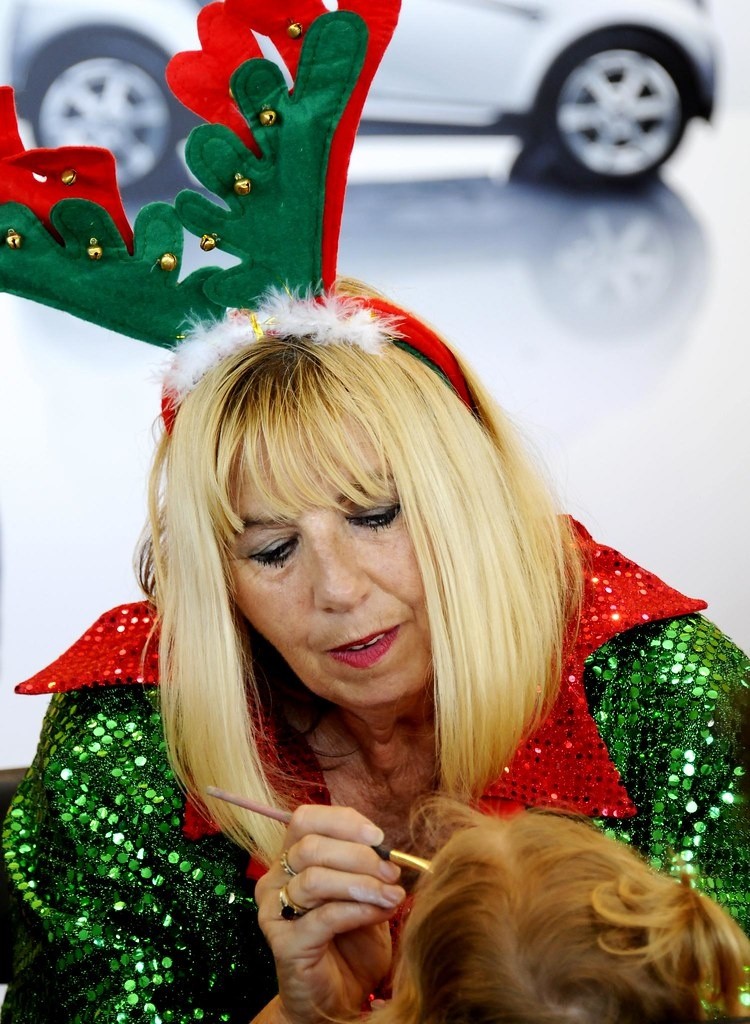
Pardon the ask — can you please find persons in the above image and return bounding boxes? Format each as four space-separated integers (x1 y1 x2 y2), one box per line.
341 795 750 1024
1 0 750 1024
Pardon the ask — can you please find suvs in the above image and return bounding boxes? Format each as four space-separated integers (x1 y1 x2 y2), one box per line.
1 0 725 212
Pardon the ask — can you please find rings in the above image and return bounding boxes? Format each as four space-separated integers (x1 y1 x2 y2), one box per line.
279 848 298 877
278 884 315 922
